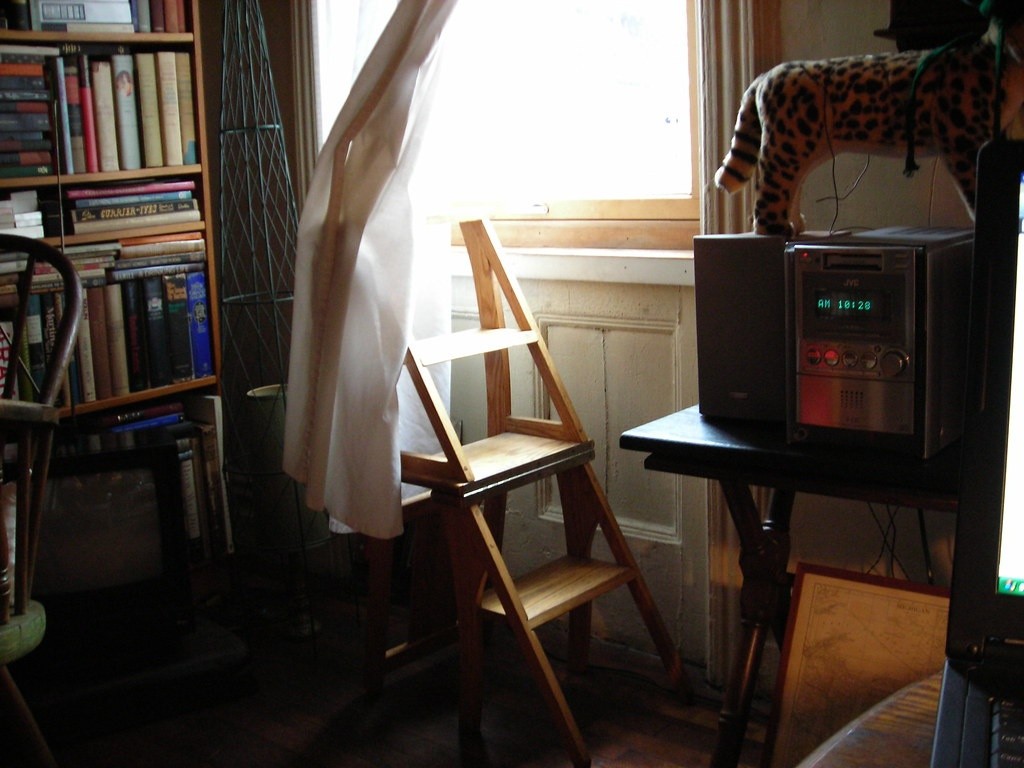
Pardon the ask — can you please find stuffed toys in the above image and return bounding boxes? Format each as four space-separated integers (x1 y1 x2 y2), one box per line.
712 1 1024 238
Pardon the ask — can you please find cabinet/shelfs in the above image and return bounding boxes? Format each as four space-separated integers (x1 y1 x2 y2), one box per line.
0 0 236 607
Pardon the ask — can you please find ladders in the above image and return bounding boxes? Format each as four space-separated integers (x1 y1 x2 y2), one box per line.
361 219 695 768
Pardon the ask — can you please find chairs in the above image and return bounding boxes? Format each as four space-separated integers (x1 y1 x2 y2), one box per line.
0 233 85 768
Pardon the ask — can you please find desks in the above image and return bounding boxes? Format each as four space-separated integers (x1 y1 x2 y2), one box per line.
620 405 953 768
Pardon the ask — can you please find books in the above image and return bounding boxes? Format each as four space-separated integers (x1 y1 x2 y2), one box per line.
0 0 193 34
0 40 199 177
0 181 212 407
0 397 237 613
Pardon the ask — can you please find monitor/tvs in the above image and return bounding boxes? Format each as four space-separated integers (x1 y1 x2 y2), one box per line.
6 442 195 657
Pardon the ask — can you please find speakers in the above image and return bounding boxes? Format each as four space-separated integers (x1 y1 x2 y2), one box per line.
694 236 791 420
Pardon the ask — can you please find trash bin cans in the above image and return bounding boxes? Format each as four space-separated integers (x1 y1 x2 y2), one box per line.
246 384 332 553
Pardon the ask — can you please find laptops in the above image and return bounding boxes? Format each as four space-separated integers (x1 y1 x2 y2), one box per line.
929 137 1024 768
